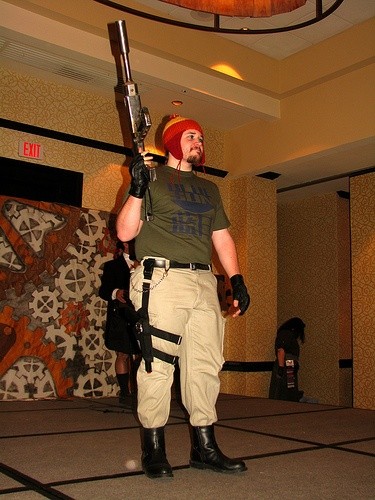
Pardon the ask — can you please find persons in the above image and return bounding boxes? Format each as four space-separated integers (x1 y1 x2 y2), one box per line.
268 317 305 400
116 114 250 479
100 240 140 405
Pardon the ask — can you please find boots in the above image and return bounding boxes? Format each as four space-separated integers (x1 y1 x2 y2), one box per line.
142 426 175 477
190 424 248 473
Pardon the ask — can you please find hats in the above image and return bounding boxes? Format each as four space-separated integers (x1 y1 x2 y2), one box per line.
161 114 206 164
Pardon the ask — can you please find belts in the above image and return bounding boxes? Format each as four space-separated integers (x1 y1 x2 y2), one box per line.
143 258 210 270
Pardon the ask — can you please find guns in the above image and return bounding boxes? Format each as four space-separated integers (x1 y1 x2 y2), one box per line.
114 19 157 221
